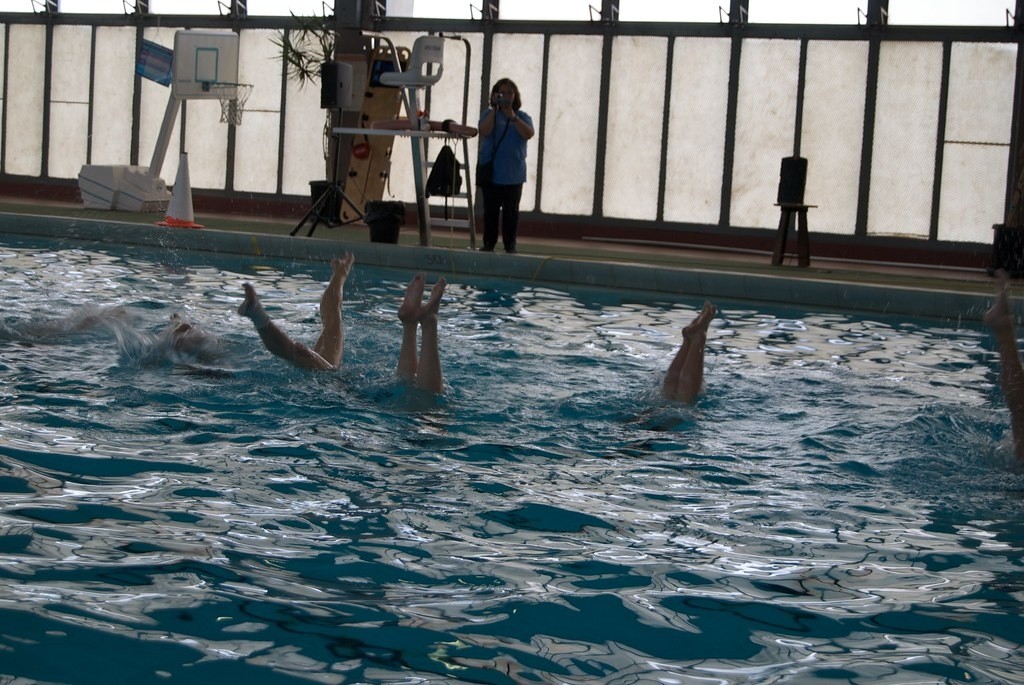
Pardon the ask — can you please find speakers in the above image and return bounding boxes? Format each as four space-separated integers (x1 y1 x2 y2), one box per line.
333 0 385 33
320 61 353 109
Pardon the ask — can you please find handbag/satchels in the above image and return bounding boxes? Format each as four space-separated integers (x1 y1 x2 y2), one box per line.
476 163 493 187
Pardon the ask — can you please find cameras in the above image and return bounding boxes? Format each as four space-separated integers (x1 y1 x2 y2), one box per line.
496 94 512 105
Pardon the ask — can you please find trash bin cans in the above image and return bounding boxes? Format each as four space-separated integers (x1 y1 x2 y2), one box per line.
364 199 404 244
309 178 337 219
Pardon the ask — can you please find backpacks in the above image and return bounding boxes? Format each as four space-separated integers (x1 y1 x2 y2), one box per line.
424 138 461 197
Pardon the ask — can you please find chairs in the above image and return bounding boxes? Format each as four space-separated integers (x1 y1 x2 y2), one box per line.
380 35 445 130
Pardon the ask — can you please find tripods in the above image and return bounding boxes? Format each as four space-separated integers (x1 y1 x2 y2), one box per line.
290 109 365 238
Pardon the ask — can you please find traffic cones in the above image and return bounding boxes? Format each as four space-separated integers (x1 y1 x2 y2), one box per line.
155 153 207 228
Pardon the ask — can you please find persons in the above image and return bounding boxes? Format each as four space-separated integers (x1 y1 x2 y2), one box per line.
982 267 1024 466
395 273 450 392
663 300 717 410
474 76 534 255
234 253 355 371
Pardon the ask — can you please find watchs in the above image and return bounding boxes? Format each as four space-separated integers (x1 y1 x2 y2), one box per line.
511 115 520 122
